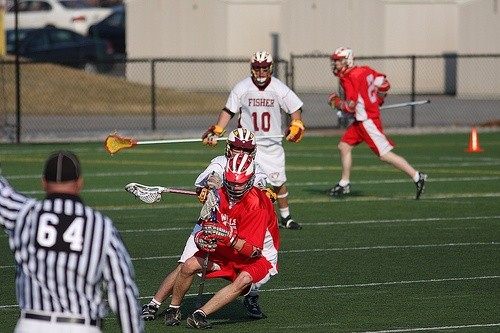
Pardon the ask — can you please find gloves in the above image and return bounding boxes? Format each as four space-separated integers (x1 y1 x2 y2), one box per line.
193 220 238 253
202 125 226 147
327 93 340 109
284 121 304 143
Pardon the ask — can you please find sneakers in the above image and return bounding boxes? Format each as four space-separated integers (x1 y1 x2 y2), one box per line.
279 215 302 230
163 307 183 326
141 303 158 320
244 294 263 318
186 312 212 328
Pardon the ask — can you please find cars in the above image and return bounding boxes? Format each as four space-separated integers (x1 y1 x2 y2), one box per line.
5 0 113 38
87 10 126 73
6 29 116 75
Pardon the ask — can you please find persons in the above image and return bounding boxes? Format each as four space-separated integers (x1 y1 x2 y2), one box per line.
327 48 428 200
202 51 304 230
0 150 145 333
141 128 280 328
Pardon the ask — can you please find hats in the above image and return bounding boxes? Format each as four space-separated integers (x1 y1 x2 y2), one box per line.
43 151 82 181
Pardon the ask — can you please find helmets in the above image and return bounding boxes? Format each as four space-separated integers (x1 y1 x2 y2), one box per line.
330 47 355 77
225 128 257 159
222 154 256 201
251 51 274 87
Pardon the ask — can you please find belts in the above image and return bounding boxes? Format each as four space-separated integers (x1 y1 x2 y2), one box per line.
19 311 96 326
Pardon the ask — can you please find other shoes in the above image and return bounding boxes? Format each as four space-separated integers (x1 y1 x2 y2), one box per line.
415 172 427 199
325 183 352 196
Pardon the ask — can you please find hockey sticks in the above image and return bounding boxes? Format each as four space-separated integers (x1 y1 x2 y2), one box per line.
336 99 432 118
123 182 198 207
196 190 221 308
103 128 286 156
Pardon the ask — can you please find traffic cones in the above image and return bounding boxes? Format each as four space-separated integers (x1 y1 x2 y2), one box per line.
463 127 483 153
